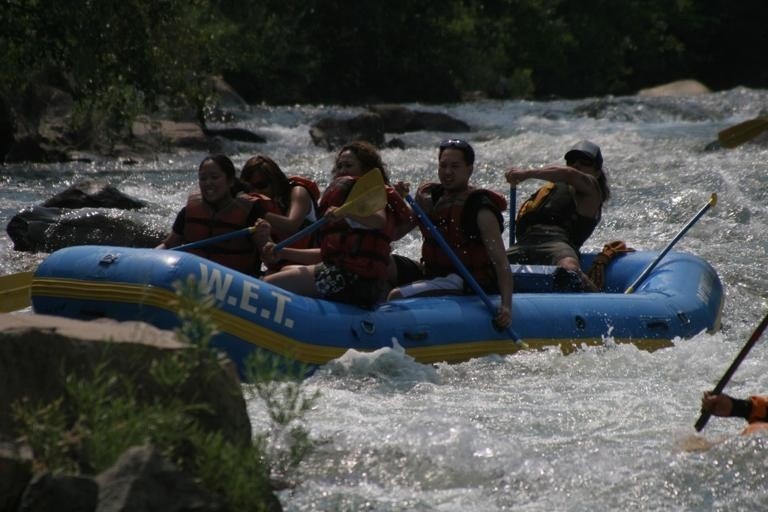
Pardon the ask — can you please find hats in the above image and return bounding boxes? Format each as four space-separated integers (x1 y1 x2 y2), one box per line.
564 139 604 164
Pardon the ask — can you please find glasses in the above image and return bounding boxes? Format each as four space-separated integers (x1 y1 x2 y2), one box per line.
439 138 466 148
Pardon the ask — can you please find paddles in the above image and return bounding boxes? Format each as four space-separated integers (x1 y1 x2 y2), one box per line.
1 223 263 315
274 166 386 254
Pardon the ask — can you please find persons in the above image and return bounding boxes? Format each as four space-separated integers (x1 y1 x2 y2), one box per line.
242 156 319 271
506 140 611 294
264 141 393 307
701 390 768 435
387 137 513 330
152 151 276 279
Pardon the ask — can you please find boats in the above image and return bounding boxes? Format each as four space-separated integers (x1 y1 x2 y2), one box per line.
30 237 722 382
